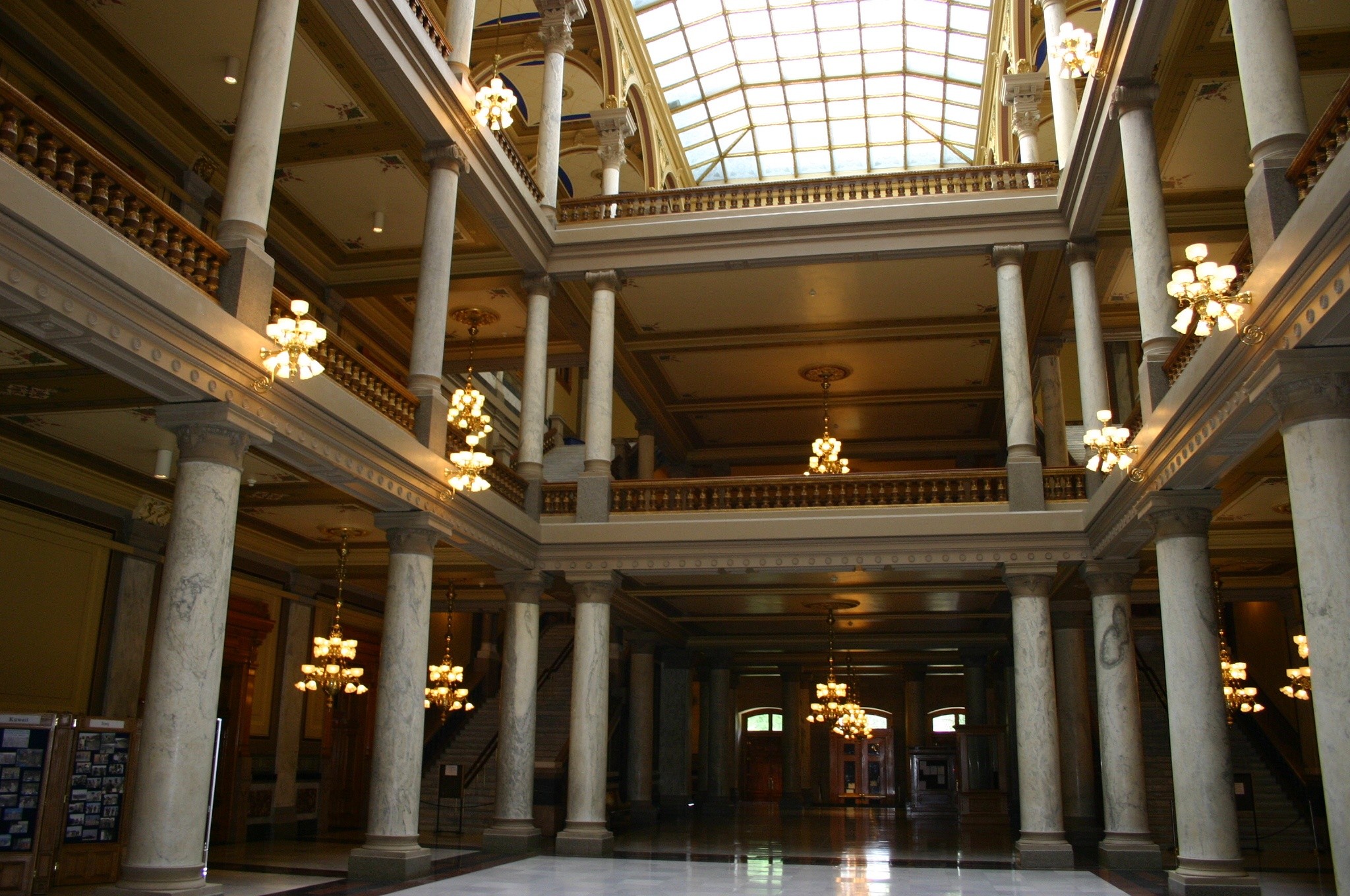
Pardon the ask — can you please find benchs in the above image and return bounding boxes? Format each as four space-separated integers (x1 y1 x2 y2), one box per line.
606 785 658 834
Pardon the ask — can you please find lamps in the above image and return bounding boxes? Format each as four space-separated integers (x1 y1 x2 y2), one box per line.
1082 409 1148 484
224 56 240 85
1212 567 1265 725
252 297 328 392
1278 625 1315 701
424 578 476 726
1054 22 1110 83
294 527 370 712
802 599 874 741
439 304 499 500
466 0 517 131
802 364 851 477
154 449 172 479
1167 242 1270 347
372 211 384 234
